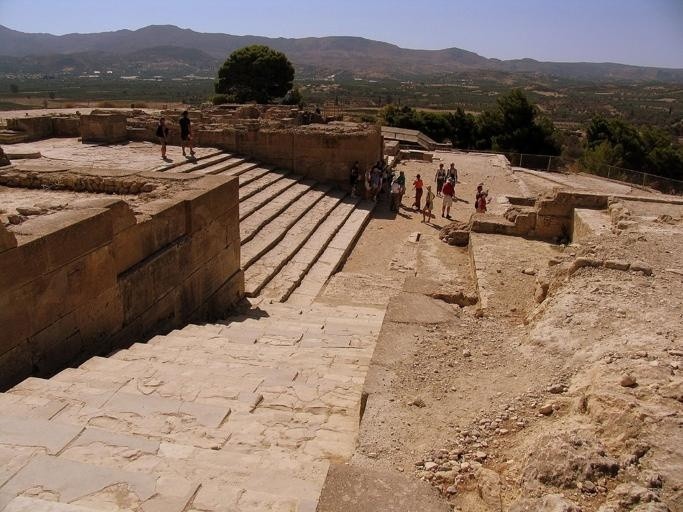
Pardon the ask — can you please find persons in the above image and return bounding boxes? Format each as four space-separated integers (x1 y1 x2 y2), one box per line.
475 185 489 214
442 178 456 219
180 111 196 155
157 118 168 159
350 160 406 213
435 161 459 197
423 185 435 223
413 174 423 212
